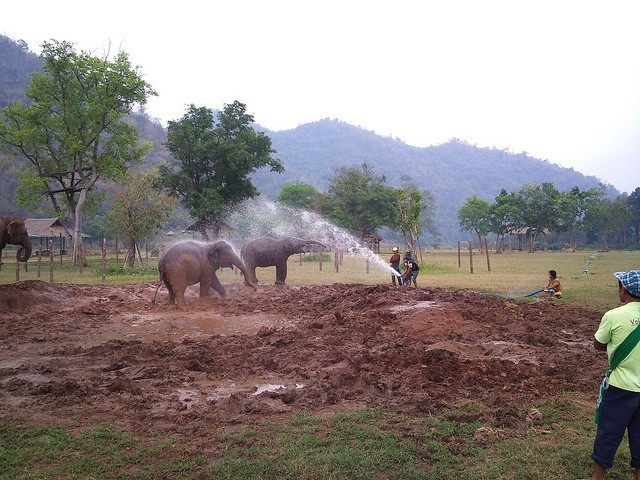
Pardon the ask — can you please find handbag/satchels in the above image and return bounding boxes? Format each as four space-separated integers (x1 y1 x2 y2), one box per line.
594 370 609 425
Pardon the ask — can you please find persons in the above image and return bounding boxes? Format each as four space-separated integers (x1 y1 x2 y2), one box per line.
400 256 419 288
389 246 403 286
586 270 640 480
542 270 562 299
404 250 411 286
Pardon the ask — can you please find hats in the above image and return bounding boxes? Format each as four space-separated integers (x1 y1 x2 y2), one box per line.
614 270 640 297
392 246 399 253
402 257 409 261
404 249 412 257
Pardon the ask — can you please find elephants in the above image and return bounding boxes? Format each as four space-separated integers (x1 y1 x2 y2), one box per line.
237 238 326 285
153 241 259 307
0 216 32 269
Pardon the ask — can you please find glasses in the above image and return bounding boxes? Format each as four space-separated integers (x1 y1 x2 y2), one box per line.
405 251 411 255
393 251 397 253
